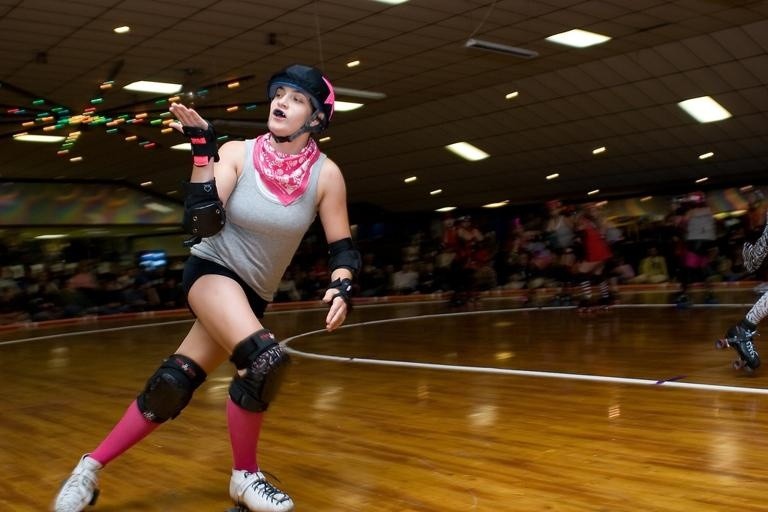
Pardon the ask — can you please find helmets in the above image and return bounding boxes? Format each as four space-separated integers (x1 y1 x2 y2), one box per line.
267 64 335 133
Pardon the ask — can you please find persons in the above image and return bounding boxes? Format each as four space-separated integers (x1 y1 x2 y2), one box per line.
724 226 767 370
51 64 361 511
2 259 190 327
273 183 767 314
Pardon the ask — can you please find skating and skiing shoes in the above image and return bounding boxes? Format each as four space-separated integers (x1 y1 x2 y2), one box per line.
520 291 623 314
669 290 718 310
227 467 295 512
54 452 104 512
714 317 761 371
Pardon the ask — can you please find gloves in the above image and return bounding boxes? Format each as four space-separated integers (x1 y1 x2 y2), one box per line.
326 276 354 314
181 119 220 167
